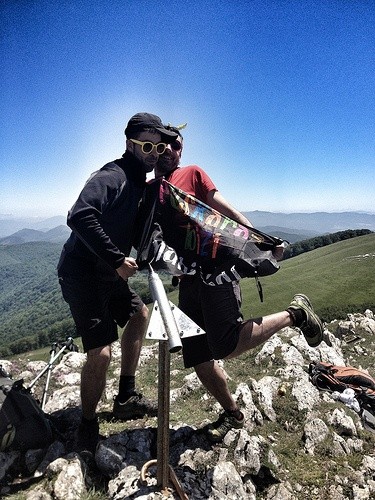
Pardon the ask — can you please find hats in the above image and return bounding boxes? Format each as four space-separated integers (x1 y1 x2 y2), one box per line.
125 112 178 141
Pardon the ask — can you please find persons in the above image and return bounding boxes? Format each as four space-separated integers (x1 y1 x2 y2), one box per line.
56 113 178 456
143 122 325 444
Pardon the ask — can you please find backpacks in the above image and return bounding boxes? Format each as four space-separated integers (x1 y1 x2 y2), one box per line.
308 360 375 406
0 378 65 451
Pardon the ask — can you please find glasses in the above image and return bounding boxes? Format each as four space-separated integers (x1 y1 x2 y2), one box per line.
169 140 183 150
130 139 167 155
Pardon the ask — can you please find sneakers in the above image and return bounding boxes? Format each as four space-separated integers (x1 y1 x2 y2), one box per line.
287 294 324 347
75 421 99 461
202 410 244 442
113 391 158 420
